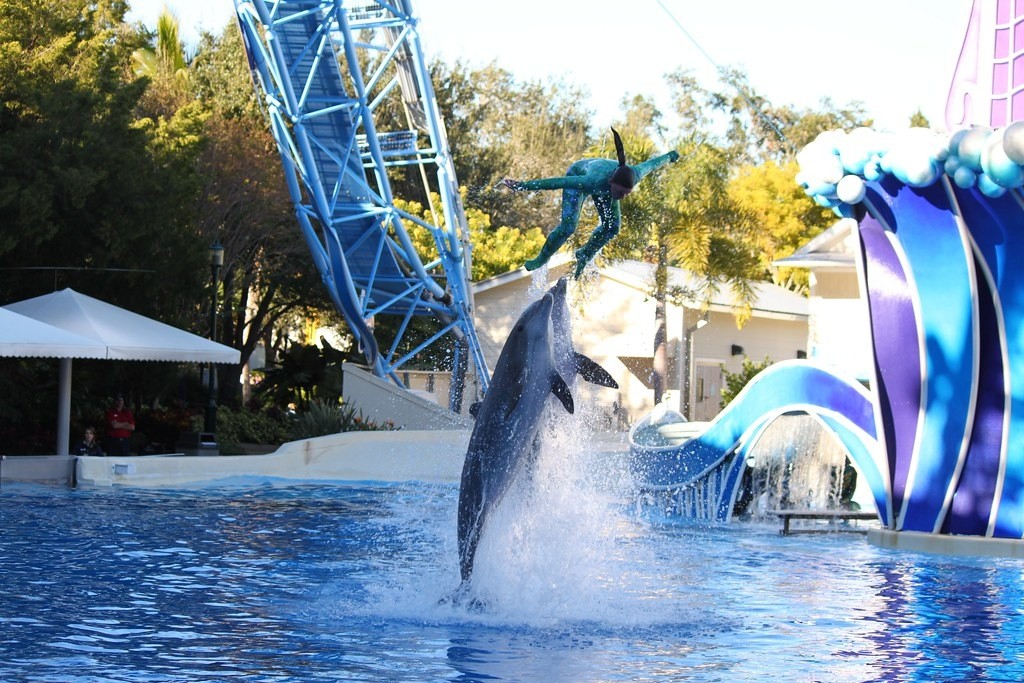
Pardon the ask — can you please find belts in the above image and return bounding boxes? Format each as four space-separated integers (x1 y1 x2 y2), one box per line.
111 438 130 441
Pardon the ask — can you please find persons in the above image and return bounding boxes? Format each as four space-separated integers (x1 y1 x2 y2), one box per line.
105 393 135 456
493 127 680 281
71 427 104 457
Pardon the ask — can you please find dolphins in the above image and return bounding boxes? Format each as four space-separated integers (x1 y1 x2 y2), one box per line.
432 277 624 615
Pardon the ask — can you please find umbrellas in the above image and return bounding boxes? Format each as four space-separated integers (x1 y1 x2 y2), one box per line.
0 288 241 457
